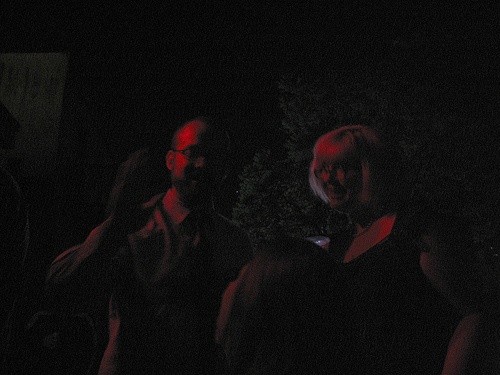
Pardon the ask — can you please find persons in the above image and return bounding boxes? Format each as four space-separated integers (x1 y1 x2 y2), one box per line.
0 161 31 375
210 236 369 374
97 114 248 374
24 252 104 375
304 124 486 375
41 145 169 373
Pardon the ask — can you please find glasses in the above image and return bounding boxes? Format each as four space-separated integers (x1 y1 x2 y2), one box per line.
171 148 208 161
